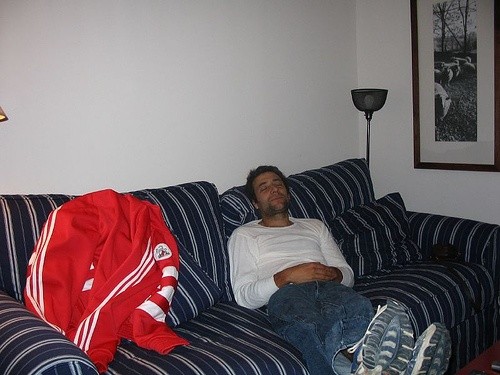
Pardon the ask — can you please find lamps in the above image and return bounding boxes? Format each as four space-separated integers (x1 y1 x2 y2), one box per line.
351 88 389 167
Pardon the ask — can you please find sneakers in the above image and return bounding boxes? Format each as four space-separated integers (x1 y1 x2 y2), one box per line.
348 300 451 375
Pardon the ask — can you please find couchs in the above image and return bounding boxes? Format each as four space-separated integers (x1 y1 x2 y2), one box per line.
0 159 500 375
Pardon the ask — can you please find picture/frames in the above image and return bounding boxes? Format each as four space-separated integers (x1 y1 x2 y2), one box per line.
410 0 500 172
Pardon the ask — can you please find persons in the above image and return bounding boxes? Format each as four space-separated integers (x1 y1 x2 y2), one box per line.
228 165 451 375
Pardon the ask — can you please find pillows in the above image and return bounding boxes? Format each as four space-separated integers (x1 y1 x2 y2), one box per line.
167 225 224 327
329 193 423 276
